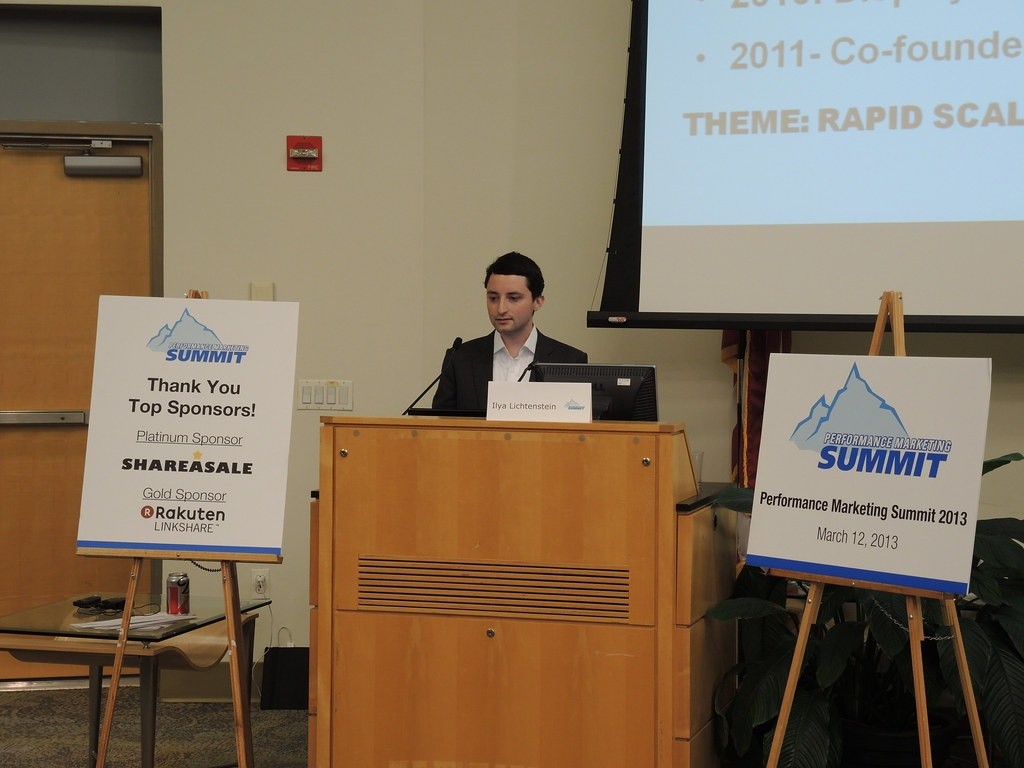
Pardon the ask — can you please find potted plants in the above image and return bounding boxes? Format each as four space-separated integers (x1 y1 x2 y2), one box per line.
698 451 1024 768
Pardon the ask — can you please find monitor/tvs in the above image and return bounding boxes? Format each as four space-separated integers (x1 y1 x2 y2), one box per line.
531 362 658 424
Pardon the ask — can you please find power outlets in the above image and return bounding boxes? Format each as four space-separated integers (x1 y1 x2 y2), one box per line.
251 569 269 596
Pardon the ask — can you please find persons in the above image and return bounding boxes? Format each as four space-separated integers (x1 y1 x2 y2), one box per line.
431 252 588 414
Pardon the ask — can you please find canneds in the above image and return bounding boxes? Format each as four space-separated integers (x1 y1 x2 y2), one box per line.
166 572 190 615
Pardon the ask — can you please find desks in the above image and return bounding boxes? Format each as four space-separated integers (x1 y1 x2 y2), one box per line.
0 591 271 768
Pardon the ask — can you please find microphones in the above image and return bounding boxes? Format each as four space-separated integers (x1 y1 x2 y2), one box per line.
402 336 462 415
